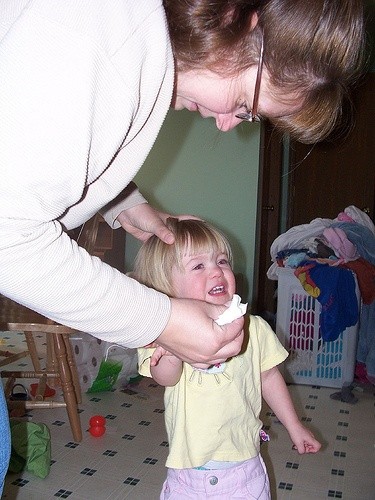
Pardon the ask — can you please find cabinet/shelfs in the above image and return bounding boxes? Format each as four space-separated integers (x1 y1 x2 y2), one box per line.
66 217 126 273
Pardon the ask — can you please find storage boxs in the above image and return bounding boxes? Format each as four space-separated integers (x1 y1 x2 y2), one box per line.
276 266 361 388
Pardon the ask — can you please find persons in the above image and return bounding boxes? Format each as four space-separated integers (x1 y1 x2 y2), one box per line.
0 0 362 499
132 218 321 500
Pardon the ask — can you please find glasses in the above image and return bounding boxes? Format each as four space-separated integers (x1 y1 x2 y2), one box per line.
235 25 266 123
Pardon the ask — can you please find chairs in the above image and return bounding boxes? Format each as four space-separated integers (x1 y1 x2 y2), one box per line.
0 213 99 442
